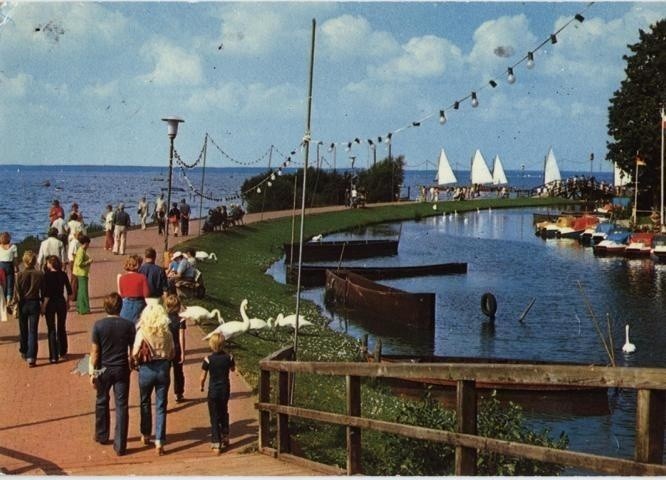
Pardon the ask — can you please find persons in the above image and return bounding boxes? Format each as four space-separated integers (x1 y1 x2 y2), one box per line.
345 186 364 208
117 254 149 367
200 333 235 454
163 294 187 404
138 247 169 303
165 247 197 296
536 174 613 198
101 204 115 250
501 185 506 198
200 204 243 233
133 303 176 456
154 193 191 236
0 199 94 367
446 185 480 201
138 196 149 230
422 185 439 202
112 203 131 256
90 291 136 456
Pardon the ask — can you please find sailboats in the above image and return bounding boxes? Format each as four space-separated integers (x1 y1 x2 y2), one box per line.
433 148 458 192
468 148 510 198
530 145 562 195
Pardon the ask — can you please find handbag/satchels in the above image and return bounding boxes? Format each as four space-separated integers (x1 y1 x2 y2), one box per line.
127 340 157 371
13 303 19 320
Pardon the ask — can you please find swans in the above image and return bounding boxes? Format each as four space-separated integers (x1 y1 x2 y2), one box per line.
177 305 225 327
620 323 636 354
202 299 251 350
272 312 316 334
311 234 321 242
195 251 219 264
247 316 275 337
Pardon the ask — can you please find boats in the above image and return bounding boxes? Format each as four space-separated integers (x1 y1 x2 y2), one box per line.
282 238 401 265
325 268 437 334
284 261 468 289
537 213 666 260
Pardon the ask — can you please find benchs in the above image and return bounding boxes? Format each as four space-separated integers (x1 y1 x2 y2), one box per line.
199 207 246 232
174 268 206 300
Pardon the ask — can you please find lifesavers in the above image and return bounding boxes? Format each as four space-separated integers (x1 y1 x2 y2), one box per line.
481 292 496 316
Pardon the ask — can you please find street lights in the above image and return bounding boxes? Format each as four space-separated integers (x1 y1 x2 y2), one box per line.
160 115 185 251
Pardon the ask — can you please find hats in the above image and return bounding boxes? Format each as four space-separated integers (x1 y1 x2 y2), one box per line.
170 251 181 260
